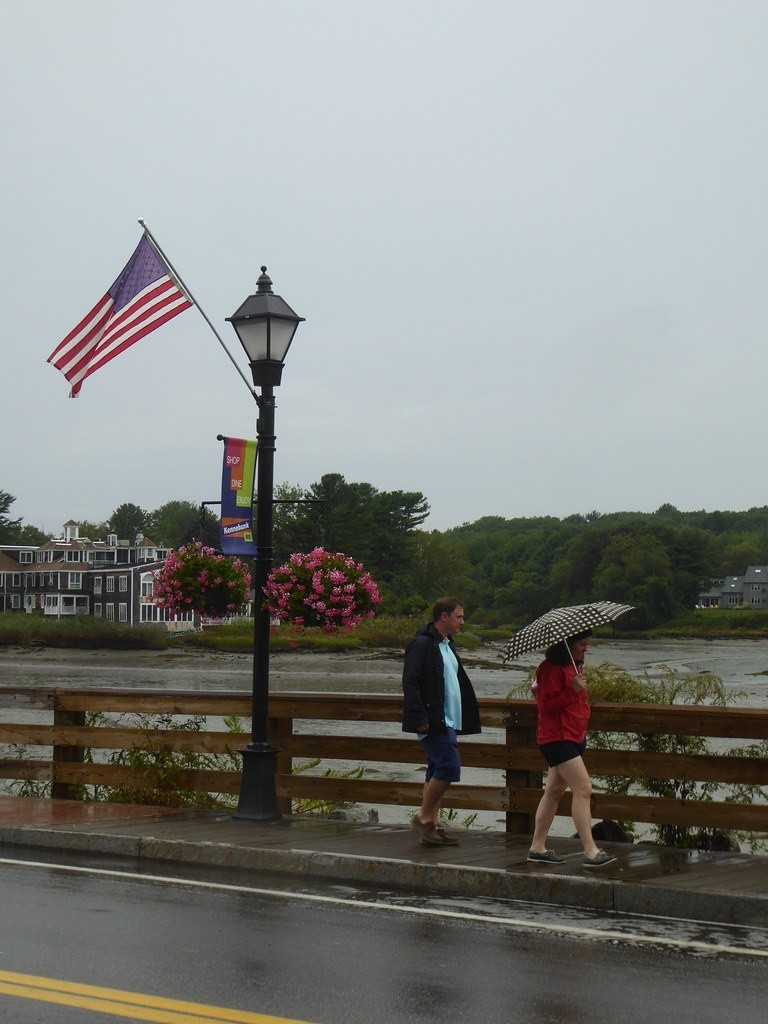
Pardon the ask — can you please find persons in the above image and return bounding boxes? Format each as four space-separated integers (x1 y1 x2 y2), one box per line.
401 596 482 847
527 628 617 867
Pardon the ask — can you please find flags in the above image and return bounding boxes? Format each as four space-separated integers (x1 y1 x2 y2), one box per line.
46 230 196 399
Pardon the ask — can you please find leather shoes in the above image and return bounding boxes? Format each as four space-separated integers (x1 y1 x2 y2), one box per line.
420 829 460 847
411 815 450 845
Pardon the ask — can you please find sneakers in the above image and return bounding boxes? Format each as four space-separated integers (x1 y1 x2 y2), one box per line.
583 849 618 867
527 850 567 864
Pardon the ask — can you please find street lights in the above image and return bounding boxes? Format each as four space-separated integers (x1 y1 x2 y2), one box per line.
226 264 307 823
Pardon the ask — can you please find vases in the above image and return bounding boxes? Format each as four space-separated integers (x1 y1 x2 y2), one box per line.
201 603 227 617
303 606 344 626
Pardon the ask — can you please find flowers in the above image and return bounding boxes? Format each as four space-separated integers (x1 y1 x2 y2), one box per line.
145 537 252 624
259 546 382 637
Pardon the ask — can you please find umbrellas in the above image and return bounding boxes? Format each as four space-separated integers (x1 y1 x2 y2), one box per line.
496 600 636 675
